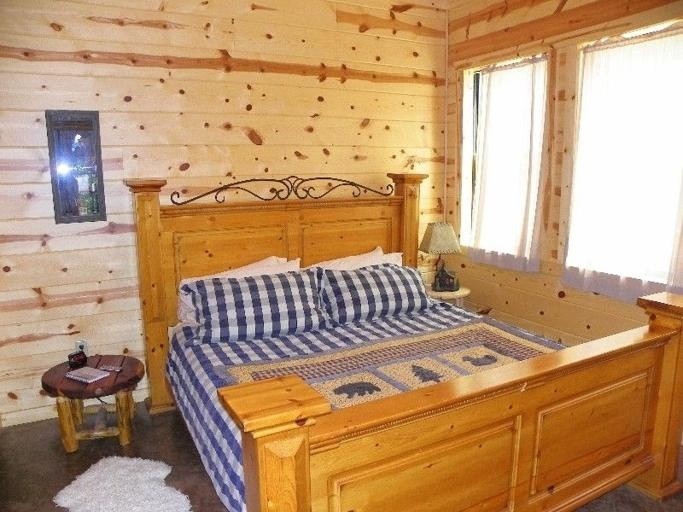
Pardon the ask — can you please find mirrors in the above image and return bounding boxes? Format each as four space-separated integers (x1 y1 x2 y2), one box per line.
44 109 106 224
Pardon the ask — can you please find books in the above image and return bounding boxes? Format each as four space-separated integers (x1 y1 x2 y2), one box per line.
66 366 110 384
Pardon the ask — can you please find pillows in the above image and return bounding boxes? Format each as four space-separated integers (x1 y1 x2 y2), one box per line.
178 245 435 340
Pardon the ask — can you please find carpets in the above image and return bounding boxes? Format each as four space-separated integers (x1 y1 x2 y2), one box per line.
51 455 191 511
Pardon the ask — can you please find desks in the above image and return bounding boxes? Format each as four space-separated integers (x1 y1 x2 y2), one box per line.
42 354 144 455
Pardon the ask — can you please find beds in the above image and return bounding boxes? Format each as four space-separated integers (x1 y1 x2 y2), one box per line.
123 172 683 511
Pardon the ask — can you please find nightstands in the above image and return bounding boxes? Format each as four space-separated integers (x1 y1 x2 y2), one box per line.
425 284 470 308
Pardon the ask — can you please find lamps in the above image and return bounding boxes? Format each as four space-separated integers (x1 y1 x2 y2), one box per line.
418 221 461 291
